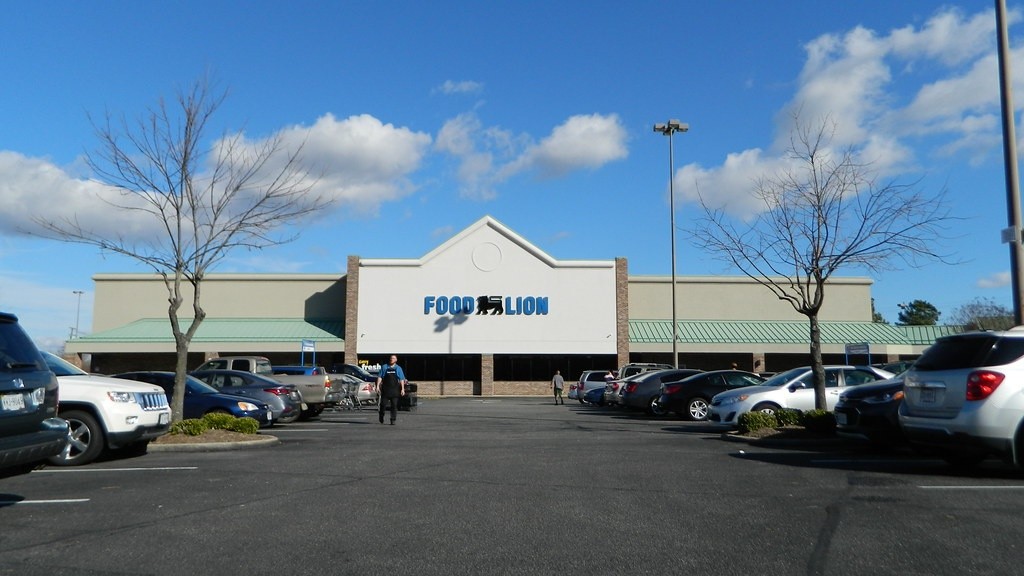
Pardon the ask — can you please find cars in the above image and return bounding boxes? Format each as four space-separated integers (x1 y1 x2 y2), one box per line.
708 365 898 438
836 367 907 455
110 371 272 426
332 373 378 405
657 369 767 422
189 369 301 429
621 370 706 420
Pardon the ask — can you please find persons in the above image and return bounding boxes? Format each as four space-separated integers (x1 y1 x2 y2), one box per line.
550 370 565 406
730 362 738 370
376 354 405 426
604 369 615 388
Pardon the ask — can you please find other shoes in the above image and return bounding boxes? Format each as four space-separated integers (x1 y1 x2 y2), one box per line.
379 415 384 424
555 399 563 405
390 420 395 425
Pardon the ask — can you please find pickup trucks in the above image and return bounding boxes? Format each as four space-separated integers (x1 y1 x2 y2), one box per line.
193 357 330 424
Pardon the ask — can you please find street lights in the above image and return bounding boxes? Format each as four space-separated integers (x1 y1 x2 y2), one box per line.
653 116 688 365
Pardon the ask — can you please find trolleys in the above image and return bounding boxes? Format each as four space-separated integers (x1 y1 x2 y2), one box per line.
330 378 362 412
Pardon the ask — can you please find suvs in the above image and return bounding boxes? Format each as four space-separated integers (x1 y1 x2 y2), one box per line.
40 350 174 467
0 312 68 479
324 364 382 407
576 371 619 408
899 329 1024 478
